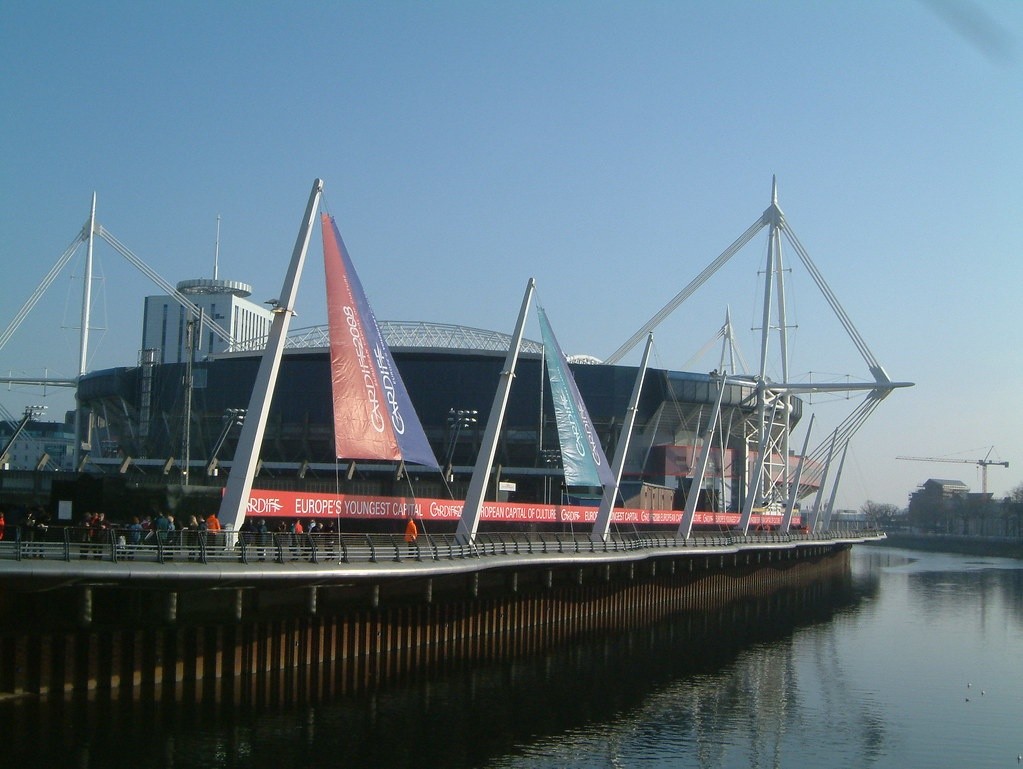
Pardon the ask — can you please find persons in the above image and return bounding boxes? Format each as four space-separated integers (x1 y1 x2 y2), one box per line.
237 518 336 563
0 512 5 539
756 524 770 533
405 516 417 558
21 511 52 559
128 512 220 562
78 513 109 559
